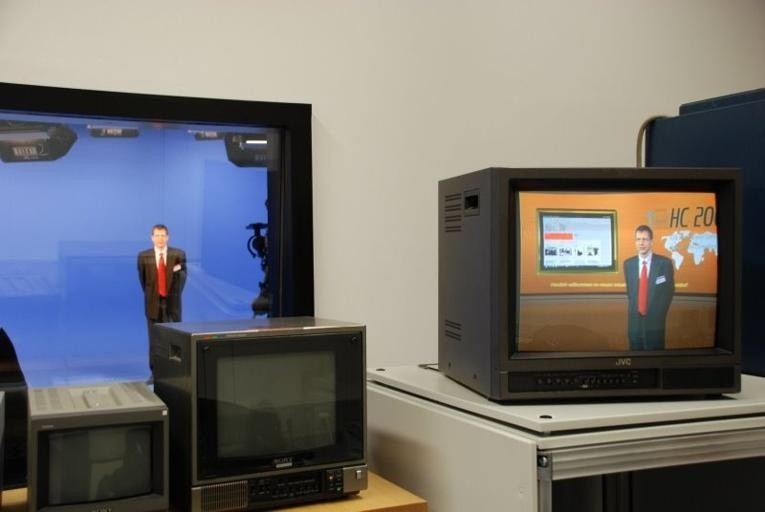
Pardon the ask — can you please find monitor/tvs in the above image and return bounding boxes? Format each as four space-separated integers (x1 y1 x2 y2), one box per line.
436 166 747 405
2 82 317 389
148 315 370 512
24 379 171 512
536 207 620 274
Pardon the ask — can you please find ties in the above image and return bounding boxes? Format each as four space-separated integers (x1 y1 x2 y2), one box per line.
638 262 648 316
157 253 167 297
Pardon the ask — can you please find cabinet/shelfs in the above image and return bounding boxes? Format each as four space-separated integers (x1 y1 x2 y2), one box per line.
0 467 429 512
365 365 765 512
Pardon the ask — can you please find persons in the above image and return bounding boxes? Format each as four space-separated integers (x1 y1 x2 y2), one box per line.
137 225 187 385
623 226 674 351
